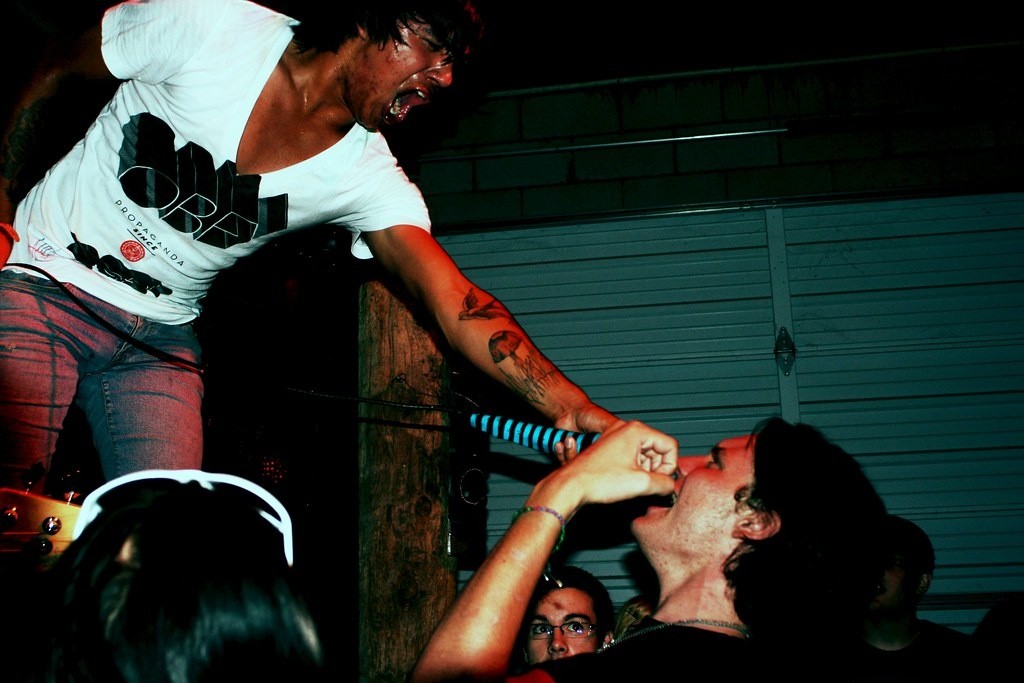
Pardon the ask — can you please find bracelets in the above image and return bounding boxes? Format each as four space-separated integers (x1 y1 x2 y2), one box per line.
510 506 565 552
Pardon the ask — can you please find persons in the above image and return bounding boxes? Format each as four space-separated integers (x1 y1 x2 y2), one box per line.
30 416 1024 683
0 1 618 495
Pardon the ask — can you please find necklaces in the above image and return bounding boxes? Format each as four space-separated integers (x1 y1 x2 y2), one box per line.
597 619 750 653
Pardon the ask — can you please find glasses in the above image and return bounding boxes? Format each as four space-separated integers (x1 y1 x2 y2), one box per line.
521 621 602 640
